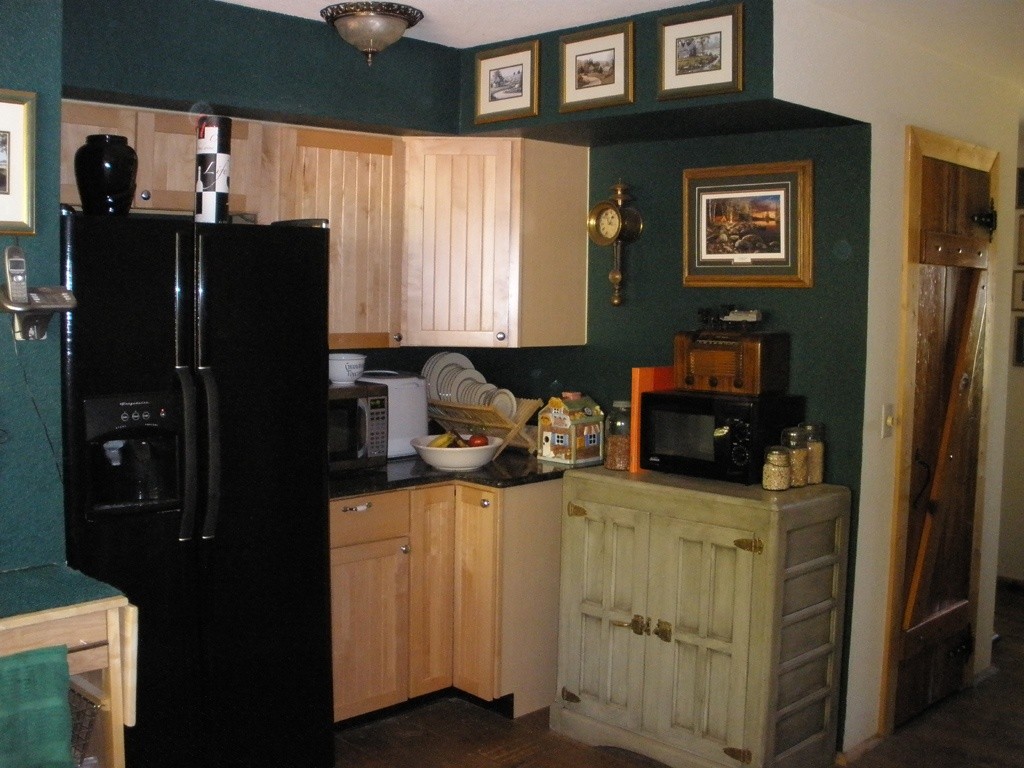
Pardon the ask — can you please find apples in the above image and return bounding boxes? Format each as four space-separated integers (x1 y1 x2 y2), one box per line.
468 432 488 447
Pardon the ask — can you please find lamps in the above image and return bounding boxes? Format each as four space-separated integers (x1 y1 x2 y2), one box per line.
319 1 424 68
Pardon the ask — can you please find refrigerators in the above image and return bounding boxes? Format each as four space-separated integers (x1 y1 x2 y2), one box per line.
59 202 335 768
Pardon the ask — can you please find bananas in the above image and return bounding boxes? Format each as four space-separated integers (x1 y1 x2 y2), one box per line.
426 434 453 448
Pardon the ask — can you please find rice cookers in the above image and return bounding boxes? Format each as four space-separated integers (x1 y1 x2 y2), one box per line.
359 370 428 459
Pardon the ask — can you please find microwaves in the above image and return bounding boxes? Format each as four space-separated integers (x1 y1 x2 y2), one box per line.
327 380 388 473
640 388 806 486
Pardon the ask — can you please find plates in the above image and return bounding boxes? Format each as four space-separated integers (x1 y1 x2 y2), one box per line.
421 351 516 421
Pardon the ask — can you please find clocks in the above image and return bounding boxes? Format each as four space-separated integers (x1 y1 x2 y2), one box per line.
587 174 644 305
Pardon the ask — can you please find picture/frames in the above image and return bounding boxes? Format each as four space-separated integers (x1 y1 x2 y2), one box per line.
681 157 817 290
0 88 38 236
473 39 542 125
558 21 636 114
656 0 744 102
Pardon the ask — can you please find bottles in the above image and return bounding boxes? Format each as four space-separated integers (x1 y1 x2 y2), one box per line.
603 400 631 471
780 427 806 488
74 134 137 214
798 422 825 485
762 445 791 490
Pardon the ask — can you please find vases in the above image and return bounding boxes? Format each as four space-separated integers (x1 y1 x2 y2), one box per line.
73 134 140 216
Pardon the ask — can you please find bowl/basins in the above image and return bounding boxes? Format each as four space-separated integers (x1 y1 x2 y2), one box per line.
408 434 504 471
328 353 367 384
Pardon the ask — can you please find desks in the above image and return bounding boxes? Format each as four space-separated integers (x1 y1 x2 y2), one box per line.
0 560 141 768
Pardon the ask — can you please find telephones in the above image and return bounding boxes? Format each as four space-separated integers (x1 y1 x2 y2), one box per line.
0 237 78 309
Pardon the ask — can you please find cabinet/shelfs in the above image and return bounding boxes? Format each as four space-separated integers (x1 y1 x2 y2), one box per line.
58 94 261 217
330 482 455 734
544 462 851 768
398 132 590 348
252 122 402 350
454 481 565 719
